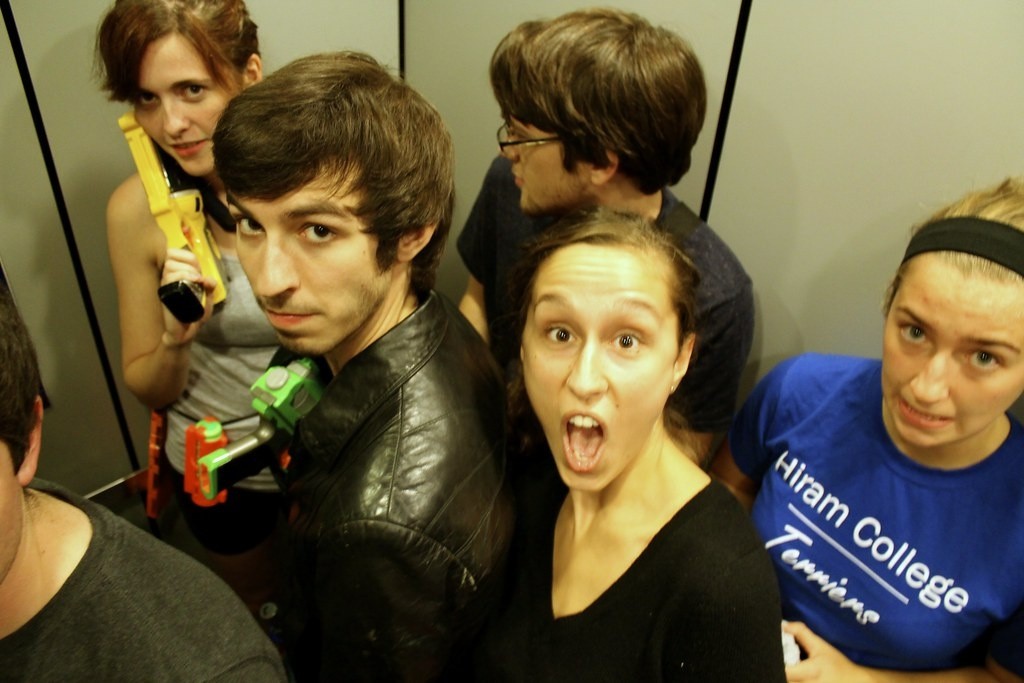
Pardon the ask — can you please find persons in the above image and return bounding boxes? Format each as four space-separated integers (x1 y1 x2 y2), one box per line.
708 179 1024 683
97 0 281 585
0 268 286 683
483 209 783 683
212 52 533 683
453 7 753 471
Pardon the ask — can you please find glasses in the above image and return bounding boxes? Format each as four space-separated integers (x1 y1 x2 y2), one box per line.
497 123 560 158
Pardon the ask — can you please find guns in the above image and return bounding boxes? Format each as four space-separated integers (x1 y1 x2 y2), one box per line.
122 110 226 327
187 357 321 516
